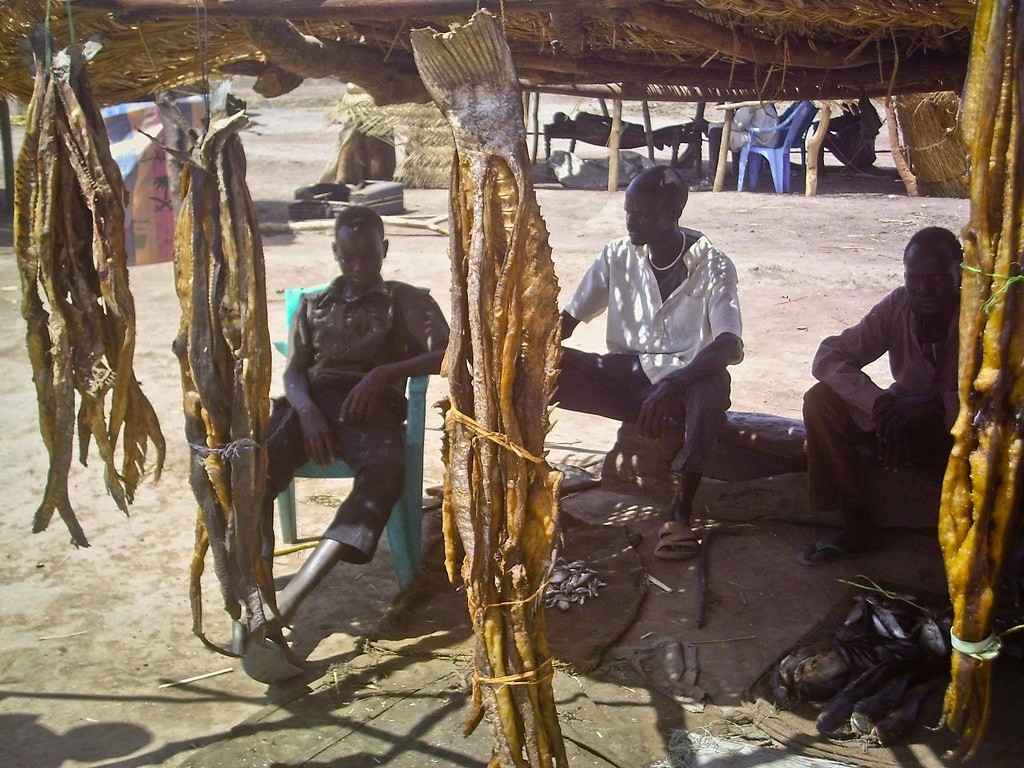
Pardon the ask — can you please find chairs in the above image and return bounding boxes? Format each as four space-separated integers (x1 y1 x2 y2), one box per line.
275 285 431 593
738 99 819 194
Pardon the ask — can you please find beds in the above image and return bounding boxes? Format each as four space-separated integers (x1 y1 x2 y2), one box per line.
544 123 703 168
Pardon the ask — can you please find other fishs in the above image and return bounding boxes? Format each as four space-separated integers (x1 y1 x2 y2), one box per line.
142 80 295 656
923 1 1024 767
410 9 569 768
11 25 167 548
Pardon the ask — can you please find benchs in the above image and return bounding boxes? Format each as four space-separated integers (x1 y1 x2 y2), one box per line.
603 407 947 530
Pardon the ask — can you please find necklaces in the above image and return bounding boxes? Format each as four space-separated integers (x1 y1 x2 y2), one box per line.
649 230 686 272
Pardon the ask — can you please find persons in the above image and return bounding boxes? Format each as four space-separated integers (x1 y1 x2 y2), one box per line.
689 104 780 192
802 225 984 566
554 112 694 140
549 165 745 560
813 101 877 170
336 122 397 186
242 206 450 683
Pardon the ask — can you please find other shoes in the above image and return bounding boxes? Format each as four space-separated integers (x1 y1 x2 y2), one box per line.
689 177 715 191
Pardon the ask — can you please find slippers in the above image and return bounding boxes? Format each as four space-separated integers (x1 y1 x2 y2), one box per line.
653 521 698 558
798 536 877 565
